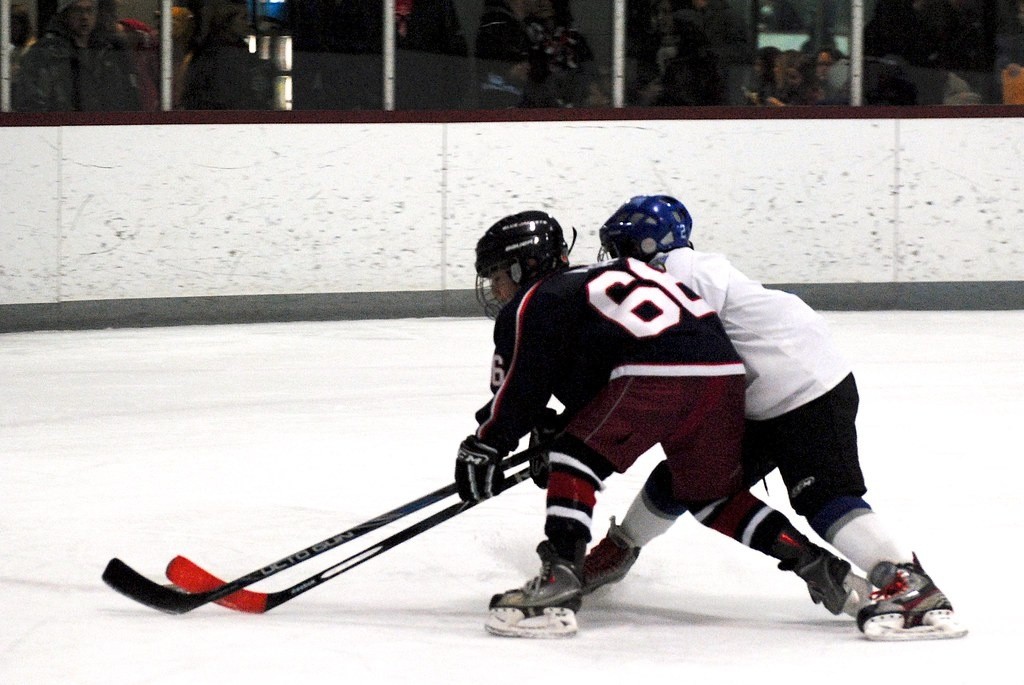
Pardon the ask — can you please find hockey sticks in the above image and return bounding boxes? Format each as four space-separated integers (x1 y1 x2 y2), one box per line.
161 461 544 619
97 442 540 620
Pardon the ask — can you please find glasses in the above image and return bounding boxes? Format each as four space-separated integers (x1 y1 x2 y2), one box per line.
67 5 98 16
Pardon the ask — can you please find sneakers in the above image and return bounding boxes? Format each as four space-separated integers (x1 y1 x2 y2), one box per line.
579 515 640 594
485 539 582 638
858 555 968 642
778 535 871 616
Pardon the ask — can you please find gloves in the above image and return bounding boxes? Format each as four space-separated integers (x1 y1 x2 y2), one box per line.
529 413 555 489
455 435 506 503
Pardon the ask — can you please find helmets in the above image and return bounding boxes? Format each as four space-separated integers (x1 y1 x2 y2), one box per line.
599 194 692 262
474 210 568 285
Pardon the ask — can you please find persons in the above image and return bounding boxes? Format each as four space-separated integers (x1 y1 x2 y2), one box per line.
578 196 969 640
455 210 872 639
1 0 1024 116
